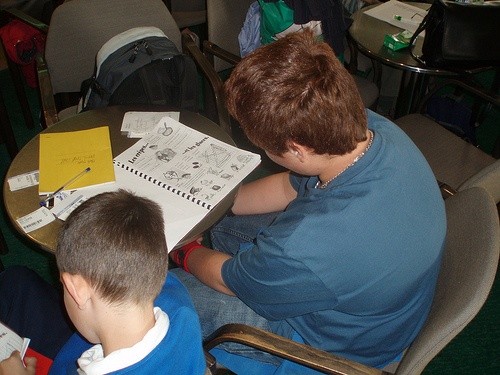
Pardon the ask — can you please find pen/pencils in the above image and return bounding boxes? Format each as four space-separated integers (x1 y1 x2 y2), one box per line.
40 167 90 207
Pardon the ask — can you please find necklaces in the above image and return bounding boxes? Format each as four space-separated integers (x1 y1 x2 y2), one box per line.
314 130 373 189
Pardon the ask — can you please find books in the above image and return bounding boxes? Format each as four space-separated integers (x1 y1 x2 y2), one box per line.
39 126 115 195
51 116 261 252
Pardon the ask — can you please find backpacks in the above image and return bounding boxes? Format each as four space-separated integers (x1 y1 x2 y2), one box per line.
238 1 262 58
77 27 187 112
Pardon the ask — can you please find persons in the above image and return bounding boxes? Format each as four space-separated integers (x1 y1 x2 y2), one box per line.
258 0 379 108
0 191 207 375
166 27 446 367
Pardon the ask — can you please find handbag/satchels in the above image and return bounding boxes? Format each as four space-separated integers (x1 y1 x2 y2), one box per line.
409 0 500 74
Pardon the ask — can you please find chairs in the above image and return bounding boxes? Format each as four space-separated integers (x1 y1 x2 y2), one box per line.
203 112 500 375
35 0 230 132
200 0 380 108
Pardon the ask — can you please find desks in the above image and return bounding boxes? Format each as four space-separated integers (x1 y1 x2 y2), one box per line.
4 105 244 256
350 2 474 122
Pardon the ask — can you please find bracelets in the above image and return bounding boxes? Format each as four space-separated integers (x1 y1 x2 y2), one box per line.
173 241 206 272
